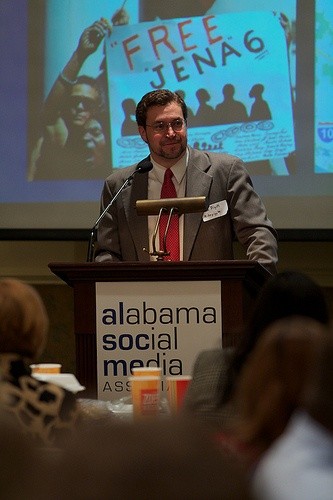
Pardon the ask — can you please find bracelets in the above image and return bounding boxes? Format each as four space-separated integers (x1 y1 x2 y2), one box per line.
59 73 78 86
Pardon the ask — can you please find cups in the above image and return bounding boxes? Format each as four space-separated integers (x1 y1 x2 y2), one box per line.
129 376 159 418
131 368 162 387
29 364 61 379
166 376 193 413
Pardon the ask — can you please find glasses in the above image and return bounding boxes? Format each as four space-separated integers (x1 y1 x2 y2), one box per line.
144 118 187 131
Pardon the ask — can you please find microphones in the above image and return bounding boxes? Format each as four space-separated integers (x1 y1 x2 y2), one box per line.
87 162 153 262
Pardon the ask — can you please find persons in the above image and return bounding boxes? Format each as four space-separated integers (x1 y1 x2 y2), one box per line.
0 270 333 500
93 90 278 271
27 6 128 183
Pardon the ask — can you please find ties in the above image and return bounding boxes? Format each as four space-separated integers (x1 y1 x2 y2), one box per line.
156 166 182 261
